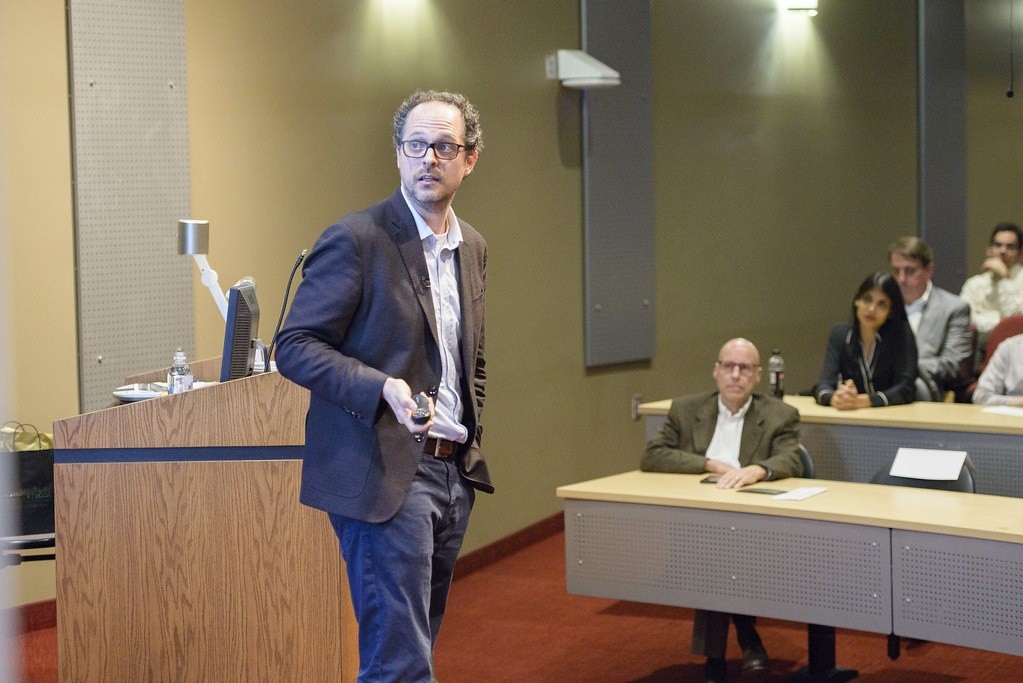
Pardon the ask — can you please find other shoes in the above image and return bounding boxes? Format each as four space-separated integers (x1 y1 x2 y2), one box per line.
742 649 768 675
704 656 726 683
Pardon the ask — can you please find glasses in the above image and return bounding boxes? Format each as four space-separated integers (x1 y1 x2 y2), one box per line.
717 360 761 378
399 137 467 161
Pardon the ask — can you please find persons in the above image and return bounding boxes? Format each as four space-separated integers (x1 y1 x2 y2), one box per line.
276 90 495 683
888 236 973 402
816 270 918 411
960 221 1023 341
639 338 804 678
970 334 1023 407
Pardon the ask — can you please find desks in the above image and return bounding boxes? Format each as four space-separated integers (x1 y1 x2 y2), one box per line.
557 468 1023 683
636 396 1023 500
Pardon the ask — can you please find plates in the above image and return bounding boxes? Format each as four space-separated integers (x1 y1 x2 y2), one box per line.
112 381 219 401
254 361 278 374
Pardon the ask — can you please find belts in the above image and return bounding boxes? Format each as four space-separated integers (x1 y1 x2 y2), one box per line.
423 438 460 459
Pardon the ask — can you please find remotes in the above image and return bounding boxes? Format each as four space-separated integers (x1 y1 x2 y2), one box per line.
412 394 431 423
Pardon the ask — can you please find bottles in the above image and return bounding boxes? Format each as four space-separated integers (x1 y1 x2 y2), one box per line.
767 349 785 399
167 352 194 394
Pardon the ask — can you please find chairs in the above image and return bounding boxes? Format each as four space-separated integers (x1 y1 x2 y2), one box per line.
871 453 974 493
966 312 1023 392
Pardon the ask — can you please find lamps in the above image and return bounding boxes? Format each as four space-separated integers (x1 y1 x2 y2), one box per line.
548 49 623 93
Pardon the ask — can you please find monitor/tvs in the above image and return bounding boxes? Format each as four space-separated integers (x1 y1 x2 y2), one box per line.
219 280 270 383
